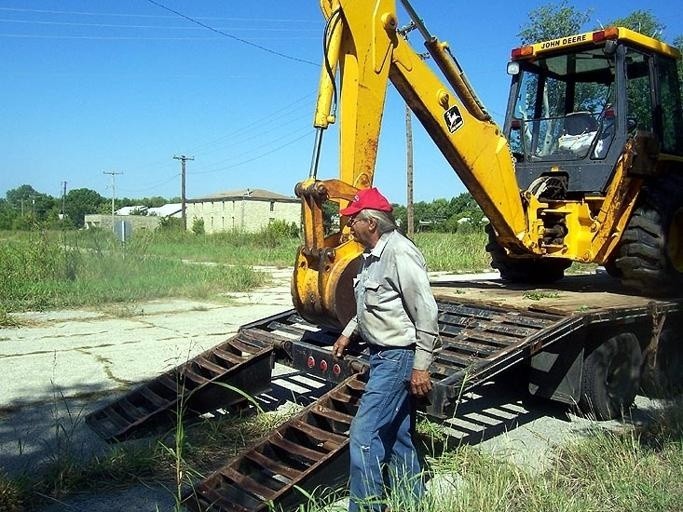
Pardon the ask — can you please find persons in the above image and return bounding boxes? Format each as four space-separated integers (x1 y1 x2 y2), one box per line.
332 188 443 512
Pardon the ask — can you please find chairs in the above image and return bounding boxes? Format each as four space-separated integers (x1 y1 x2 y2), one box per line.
564 111 596 136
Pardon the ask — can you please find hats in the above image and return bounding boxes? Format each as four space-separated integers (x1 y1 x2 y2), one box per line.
341 188 393 215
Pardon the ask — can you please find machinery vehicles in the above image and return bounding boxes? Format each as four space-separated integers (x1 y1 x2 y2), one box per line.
291 1 683 338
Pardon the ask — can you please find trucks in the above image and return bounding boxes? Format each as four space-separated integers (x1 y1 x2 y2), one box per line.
85 274 682 512
